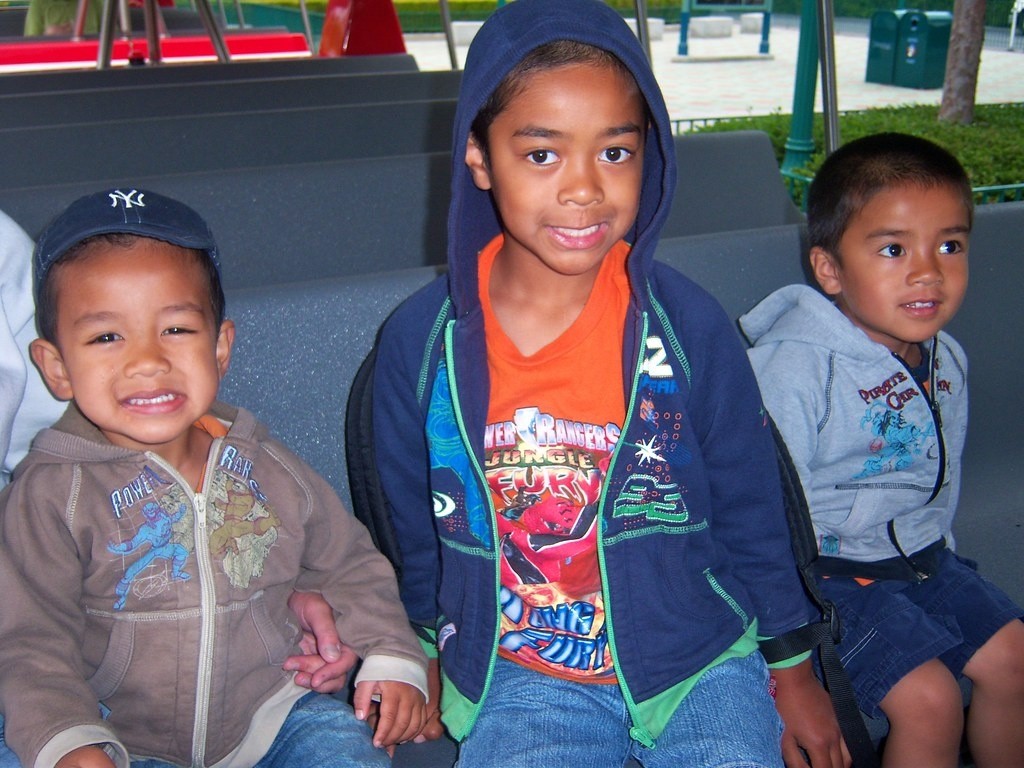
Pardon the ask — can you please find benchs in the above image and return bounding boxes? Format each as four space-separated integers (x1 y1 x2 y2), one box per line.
0 24 1024 768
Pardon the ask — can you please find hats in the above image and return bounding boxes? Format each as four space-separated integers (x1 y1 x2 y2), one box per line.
32 188 226 311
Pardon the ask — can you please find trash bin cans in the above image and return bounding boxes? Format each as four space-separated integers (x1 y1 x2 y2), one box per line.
865 8 952 89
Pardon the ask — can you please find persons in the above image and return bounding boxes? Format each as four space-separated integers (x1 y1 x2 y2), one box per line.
740 130 1024 768
370 2 851 768
0 187 429 768
